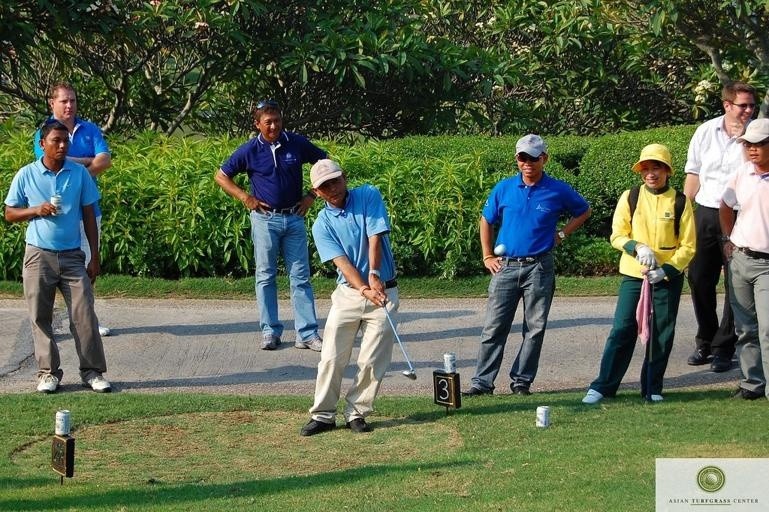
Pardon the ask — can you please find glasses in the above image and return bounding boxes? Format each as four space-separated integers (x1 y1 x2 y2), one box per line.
727 101 757 109
741 139 769 149
517 153 545 163
254 100 283 119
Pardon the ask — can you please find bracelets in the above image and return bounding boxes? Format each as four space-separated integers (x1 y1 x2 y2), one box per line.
368 270 383 278
481 255 496 262
307 190 318 200
357 286 369 295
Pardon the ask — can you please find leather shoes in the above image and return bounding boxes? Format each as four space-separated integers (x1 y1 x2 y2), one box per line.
510 381 538 394
688 346 706 366
459 379 496 397
711 354 733 371
730 380 761 399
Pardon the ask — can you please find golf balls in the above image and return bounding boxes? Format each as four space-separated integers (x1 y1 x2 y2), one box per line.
493 245 505 255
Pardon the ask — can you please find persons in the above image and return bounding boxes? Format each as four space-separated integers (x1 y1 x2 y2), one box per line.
298 157 398 437
461 132 593 399
682 80 756 373
33 86 113 337
213 100 333 351
4 124 113 394
719 118 769 400
583 143 697 403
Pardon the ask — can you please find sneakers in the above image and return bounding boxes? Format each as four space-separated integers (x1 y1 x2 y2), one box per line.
291 334 324 351
258 330 280 348
642 390 664 402
300 413 336 435
582 383 608 404
81 374 111 394
37 371 61 392
346 415 370 433
99 322 110 338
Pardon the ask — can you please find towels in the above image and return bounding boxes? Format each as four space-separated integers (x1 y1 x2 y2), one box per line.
636 275 653 347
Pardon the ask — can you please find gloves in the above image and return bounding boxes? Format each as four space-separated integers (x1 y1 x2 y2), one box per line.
644 264 667 285
634 245 659 268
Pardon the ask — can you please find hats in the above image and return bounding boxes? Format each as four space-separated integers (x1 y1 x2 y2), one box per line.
514 133 547 158
632 142 675 177
736 117 769 143
310 157 342 187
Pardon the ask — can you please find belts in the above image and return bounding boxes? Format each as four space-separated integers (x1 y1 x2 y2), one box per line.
251 202 302 215
497 252 555 267
735 245 768 260
346 281 401 293
25 238 84 255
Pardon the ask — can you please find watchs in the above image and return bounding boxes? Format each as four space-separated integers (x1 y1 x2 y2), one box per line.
719 234 732 242
556 230 568 241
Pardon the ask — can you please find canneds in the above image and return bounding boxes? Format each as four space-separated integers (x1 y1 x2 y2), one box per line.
443 352 456 373
51 195 62 216
55 410 70 435
536 406 549 427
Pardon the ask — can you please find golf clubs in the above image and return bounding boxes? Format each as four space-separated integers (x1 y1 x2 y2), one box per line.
382 301 416 380
647 270 654 401
